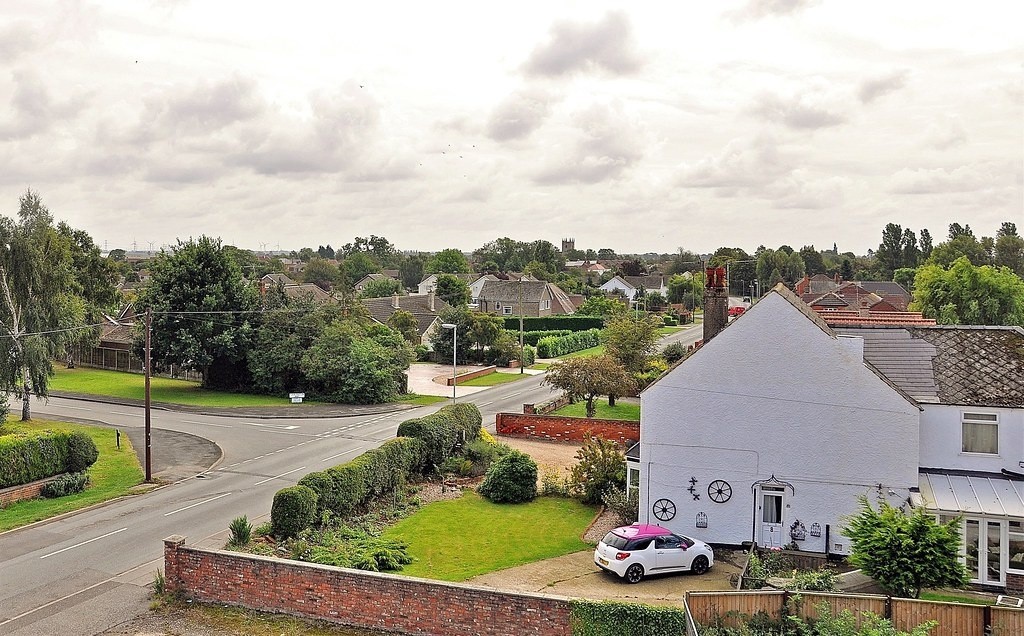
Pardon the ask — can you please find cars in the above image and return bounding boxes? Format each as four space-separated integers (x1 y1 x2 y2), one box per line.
594 522 715 583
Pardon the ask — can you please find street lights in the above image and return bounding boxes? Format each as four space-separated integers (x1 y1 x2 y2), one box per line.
629 300 638 332
740 280 744 300
442 323 456 403
